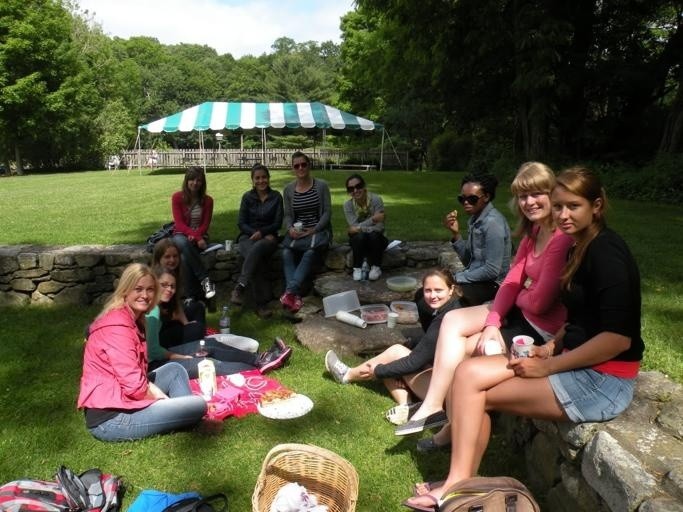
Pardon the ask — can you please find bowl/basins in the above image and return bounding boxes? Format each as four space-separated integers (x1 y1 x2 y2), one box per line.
387 275 416 292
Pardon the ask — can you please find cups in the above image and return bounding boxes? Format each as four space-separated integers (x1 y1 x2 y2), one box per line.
387 313 398 328
224 240 233 251
336 310 366 330
512 334 535 359
293 222 303 232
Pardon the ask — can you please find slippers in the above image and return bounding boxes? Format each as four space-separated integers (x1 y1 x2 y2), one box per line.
415 482 433 497
403 493 441 512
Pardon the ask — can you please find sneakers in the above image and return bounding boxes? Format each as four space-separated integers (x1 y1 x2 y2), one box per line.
368 266 382 280
201 277 216 299
255 305 274 319
230 285 245 305
280 290 294 307
269 336 286 352
257 347 291 374
324 349 350 385
352 267 362 281
394 409 449 434
416 437 452 452
386 402 423 426
288 295 305 312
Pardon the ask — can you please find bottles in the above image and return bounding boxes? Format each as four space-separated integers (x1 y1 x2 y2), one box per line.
361 258 370 281
218 306 233 333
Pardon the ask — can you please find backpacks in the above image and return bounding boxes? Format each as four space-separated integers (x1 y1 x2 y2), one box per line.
0 463 127 512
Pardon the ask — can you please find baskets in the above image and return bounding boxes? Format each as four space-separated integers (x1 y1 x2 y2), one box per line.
251 440 360 512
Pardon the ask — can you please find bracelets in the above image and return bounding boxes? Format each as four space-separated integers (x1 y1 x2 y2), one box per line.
539 343 552 361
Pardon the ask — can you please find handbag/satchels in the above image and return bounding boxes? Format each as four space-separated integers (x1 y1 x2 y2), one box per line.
128 488 229 512
439 476 541 512
145 221 176 254
281 228 330 251
348 222 385 241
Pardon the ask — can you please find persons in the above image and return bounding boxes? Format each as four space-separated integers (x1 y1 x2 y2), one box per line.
401 165 646 511
108 147 161 170
152 237 207 343
140 267 293 378
75 262 225 443
229 162 285 318
414 169 513 334
343 173 389 281
170 165 216 306
393 161 577 454
324 265 464 426
279 150 333 313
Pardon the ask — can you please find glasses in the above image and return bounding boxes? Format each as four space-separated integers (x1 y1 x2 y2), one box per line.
347 183 364 193
457 194 486 206
294 162 307 169
160 282 177 289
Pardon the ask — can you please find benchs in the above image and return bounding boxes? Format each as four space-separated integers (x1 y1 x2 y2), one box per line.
328 164 376 172
